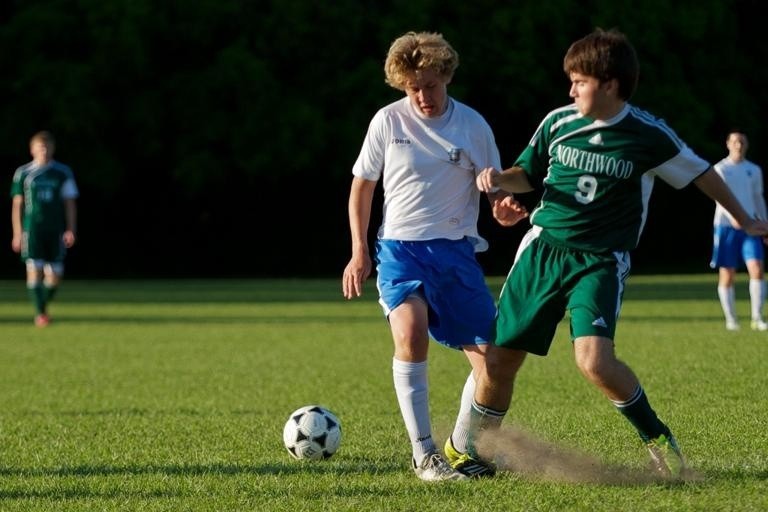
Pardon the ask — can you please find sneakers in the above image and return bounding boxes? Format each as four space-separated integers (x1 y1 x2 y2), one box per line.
646 427 685 480
412 434 495 481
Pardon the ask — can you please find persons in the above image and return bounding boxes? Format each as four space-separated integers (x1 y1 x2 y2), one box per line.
12 131 80 327
709 130 768 331
451 27 768 482
342 31 529 483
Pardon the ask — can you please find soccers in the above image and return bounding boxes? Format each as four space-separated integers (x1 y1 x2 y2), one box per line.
282 407 342 460
